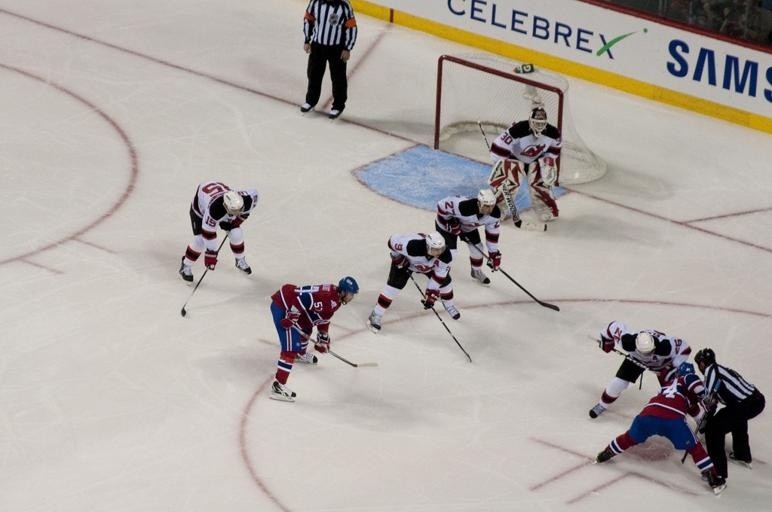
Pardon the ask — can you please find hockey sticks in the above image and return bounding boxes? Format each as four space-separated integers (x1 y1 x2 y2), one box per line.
182 232 228 316
293 327 379 368
467 238 559 311
477 121 548 231
409 275 472 364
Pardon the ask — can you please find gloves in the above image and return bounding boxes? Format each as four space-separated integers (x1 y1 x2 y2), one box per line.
314 333 334 354
394 256 411 272
280 312 301 328
445 216 462 236
486 249 503 273
696 396 718 433
421 289 440 309
232 216 243 228
204 254 217 270
599 339 614 353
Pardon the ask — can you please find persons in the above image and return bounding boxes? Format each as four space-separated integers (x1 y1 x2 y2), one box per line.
599 364 720 490
178 181 258 279
585 320 692 417
436 189 500 285
488 107 562 223
695 346 765 487
299 1 358 120
366 230 462 326
269 276 359 393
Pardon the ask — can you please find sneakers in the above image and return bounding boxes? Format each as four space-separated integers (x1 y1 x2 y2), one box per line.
234 255 253 275
368 309 382 333
270 380 297 399
470 266 491 284
596 450 612 463
441 299 461 320
702 451 752 489
299 351 318 363
589 401 607 419
180 256 194 282
300 99 345 119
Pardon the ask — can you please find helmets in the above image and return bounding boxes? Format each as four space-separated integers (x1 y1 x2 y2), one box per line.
339 277 358 294
476 188 496 215
224 192 245 217
635 332 657 357
425 230 447 257
530 107 548 134
677 347 715 375
